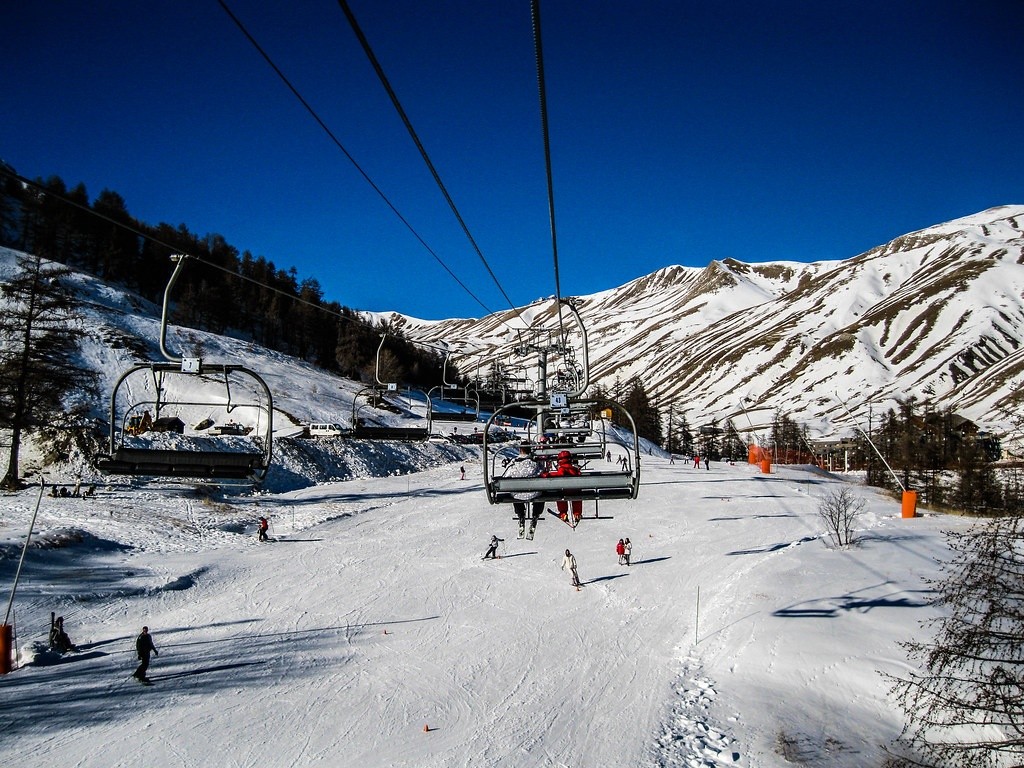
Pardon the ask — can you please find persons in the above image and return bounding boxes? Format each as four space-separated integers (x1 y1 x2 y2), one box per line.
560 549 580 585
460 466 466 480
504 441 550 540
540 450 584 522
257 525 264 541
623 538 632 565
132 626 159 683
616 539 624 564
49 616 77 655
484 535 504 558
259 517 268 540
531 413 709 475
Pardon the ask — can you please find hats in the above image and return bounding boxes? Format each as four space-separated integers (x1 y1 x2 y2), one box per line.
540 437 547 441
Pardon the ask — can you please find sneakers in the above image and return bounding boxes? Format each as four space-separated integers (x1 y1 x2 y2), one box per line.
573 514 582 522
529 515 538 534
558 512 568 522
518 515 525 535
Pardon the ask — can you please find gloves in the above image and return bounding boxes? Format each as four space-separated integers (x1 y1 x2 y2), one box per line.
138 655 141 660
155 650 158 656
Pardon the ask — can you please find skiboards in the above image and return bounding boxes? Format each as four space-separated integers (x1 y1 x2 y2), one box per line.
547 507 583 529
516 519 525 540
526 519 537 541
